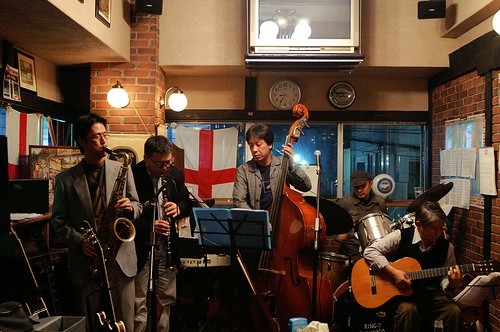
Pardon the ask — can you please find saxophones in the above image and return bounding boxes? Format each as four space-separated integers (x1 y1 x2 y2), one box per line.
81 145 137 280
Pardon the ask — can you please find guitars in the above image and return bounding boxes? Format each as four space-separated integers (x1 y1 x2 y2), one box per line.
350 255 500 310
77 218 127 332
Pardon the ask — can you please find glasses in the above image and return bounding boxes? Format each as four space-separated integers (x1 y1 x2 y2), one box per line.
147 155 174 167
421 223 448 232
81 134 110 141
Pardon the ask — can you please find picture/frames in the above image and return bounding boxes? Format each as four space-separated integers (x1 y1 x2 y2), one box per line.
17 52 36 92
95 0 111 28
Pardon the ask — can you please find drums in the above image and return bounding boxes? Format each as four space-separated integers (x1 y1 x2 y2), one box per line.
319 249 348 286
334 278 396 332
355 211 394 252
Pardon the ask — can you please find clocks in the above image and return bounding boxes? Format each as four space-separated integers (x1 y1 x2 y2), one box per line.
269 79 301 110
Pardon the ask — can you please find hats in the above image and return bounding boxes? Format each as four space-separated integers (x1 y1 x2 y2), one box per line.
350 171 371 186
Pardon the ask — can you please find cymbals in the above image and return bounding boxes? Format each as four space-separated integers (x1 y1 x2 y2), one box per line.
302 195 354 238
407 181 454 214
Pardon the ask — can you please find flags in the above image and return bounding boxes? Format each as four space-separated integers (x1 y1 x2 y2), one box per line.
48 118 71 147
6 106 41 179
175 126 238 199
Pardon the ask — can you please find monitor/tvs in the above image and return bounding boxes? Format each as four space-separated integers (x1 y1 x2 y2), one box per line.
248 0 360 53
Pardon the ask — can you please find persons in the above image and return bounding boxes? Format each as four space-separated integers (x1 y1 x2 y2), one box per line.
49 112 144 332
232 124 312 231
130 135 191 332
364 201 463 332
336 170 387 241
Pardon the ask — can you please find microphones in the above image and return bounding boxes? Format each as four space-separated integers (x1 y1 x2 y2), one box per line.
152 179 172 202
314 150 322 174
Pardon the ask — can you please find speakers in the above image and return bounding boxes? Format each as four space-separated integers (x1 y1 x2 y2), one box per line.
135 0 163 15
418 1 446 19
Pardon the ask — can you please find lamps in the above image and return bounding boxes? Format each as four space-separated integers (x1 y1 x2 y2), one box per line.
107 80 130 108
160 86 187 112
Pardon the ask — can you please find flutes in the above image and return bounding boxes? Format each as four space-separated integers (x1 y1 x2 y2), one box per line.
158 174 176 256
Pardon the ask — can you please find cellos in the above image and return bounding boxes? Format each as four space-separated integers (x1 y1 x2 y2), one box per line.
249 104 335 332
